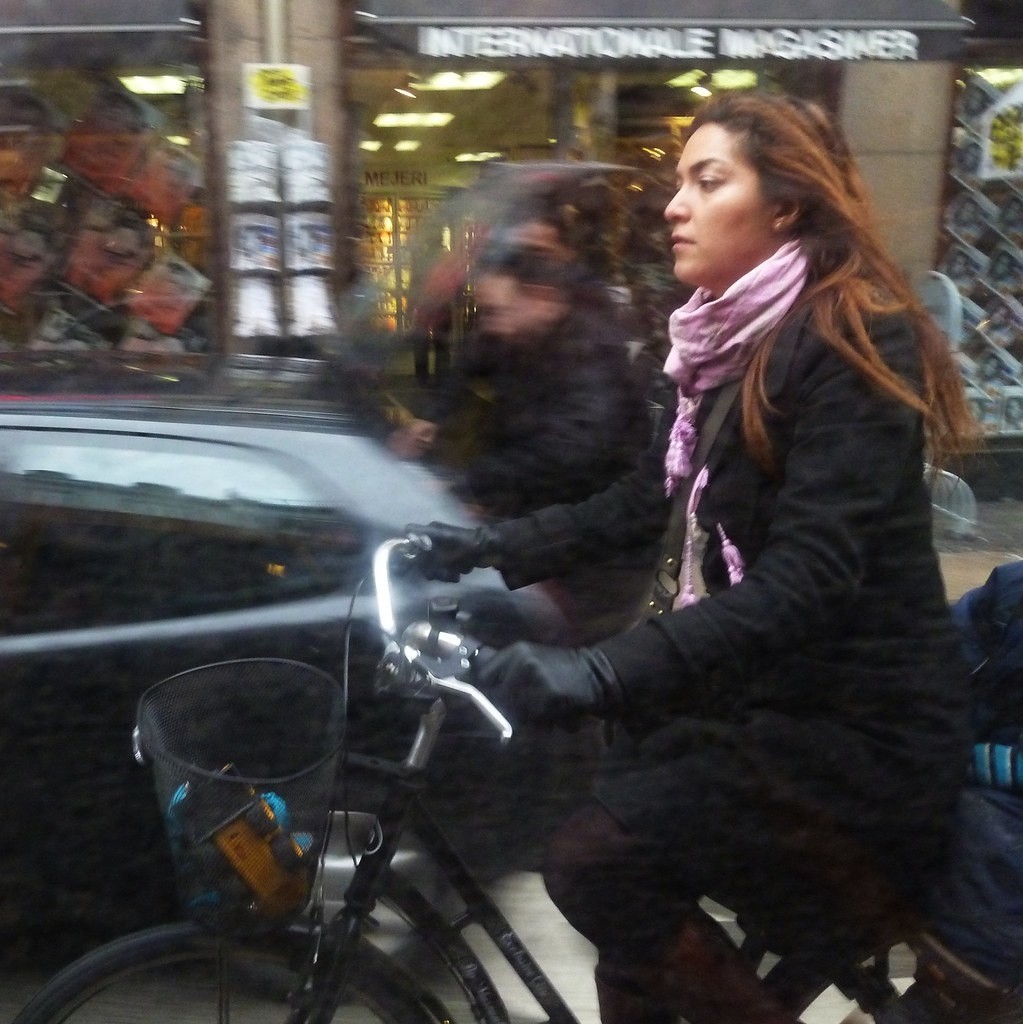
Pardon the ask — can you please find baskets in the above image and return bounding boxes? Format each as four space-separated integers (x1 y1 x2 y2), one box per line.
136 657 347 936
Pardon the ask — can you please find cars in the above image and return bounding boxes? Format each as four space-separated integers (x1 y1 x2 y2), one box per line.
0 346 589 986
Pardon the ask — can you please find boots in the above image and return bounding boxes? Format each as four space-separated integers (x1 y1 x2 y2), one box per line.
595 915 807 1024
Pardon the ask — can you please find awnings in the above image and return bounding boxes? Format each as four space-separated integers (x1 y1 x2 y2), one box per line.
352 0 984 72
0 0 205 67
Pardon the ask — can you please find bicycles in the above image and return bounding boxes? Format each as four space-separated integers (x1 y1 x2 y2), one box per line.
11 532 1022 1023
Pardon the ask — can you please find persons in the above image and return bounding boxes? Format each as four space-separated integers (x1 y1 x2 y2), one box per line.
399 88 975 1024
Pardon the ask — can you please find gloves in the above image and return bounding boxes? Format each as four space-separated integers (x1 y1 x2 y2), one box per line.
404 520 503 582
477 640 623 732
